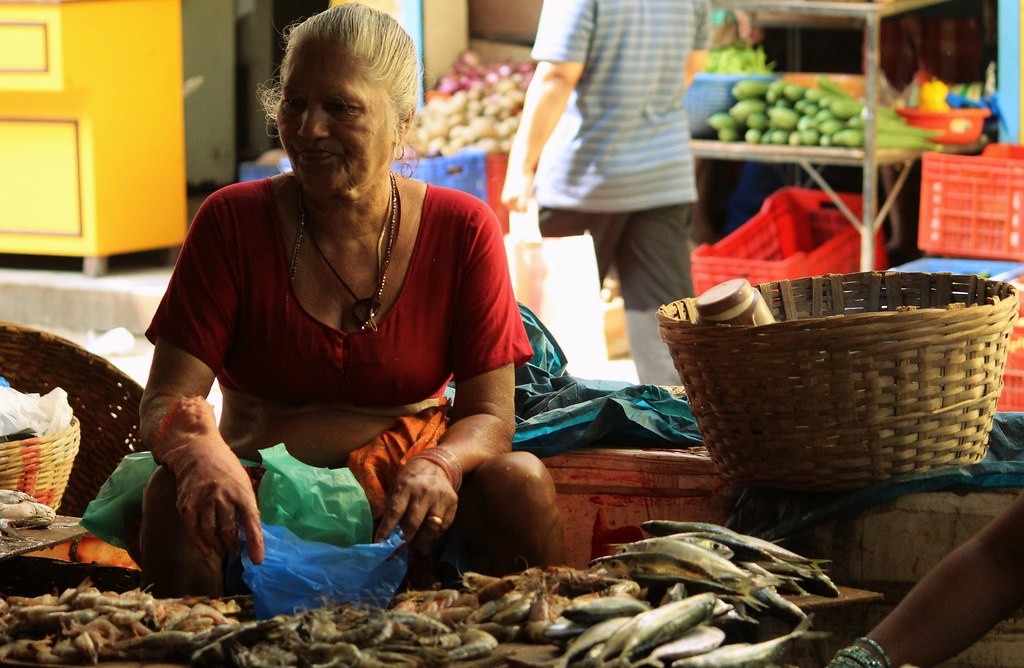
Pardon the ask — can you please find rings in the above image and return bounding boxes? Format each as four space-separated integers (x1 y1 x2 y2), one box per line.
428 516 443 526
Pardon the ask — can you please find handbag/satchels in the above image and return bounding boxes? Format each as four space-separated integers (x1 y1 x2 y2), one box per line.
499 234 608 380
241 522 410 619
256 442 374 546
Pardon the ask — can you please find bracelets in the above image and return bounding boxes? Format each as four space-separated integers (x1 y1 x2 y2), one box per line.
406 447 462 493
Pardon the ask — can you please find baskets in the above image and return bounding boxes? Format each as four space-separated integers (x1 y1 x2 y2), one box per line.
0 416 81 510
917 143 1024 262
0 321 145 517
994 274 1024 411
657 270 1024 488
690 186 887 300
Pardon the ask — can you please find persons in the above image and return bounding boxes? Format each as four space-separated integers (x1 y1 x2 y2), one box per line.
500 0 712 387
139 5 565 599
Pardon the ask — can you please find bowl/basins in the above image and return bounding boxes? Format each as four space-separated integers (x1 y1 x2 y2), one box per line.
896 106 990 144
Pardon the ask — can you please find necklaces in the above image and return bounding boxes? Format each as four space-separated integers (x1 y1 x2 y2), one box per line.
289 170 403 334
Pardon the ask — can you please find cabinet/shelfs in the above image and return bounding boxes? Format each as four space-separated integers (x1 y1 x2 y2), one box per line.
685 0 997 274
0 0 189 278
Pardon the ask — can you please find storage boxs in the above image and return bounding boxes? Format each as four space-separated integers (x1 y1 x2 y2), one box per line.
236 149 514 243
691 142 1024 414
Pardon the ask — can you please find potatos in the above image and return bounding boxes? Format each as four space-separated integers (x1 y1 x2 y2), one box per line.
402 79 527 157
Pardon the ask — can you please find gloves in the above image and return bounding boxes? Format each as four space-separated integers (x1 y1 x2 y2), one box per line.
152 396 264 564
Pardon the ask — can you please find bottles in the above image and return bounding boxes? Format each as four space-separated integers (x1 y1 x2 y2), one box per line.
694 277 777 326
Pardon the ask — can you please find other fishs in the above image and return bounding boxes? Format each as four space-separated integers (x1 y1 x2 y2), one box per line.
543 519 839 668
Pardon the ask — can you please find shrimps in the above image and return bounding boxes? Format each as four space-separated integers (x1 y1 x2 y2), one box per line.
0 568 570 668
